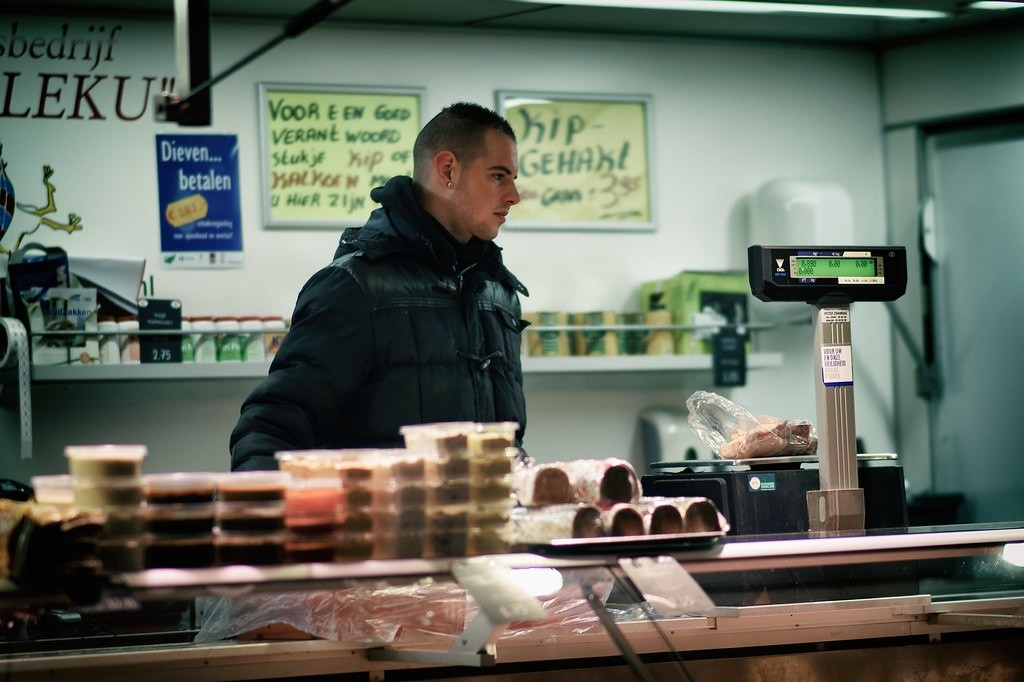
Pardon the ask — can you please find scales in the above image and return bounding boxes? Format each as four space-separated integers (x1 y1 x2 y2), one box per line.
641 245 907 539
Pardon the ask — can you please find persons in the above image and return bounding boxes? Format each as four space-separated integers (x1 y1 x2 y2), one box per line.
227 101 531 562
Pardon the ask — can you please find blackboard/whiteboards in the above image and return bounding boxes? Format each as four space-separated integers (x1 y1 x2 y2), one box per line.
496 86 655 230
257 79 423 229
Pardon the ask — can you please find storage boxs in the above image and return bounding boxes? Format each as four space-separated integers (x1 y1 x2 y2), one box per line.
33 423 523 578
7 242 100 364
139 296 181 358
644 270 753 355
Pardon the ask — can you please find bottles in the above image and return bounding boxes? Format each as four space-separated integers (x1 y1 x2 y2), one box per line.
98 316 287 365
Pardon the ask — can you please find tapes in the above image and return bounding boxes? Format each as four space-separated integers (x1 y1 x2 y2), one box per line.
0 316 33 460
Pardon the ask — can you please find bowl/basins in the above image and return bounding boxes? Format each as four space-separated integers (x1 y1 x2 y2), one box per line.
32 445 343 568
